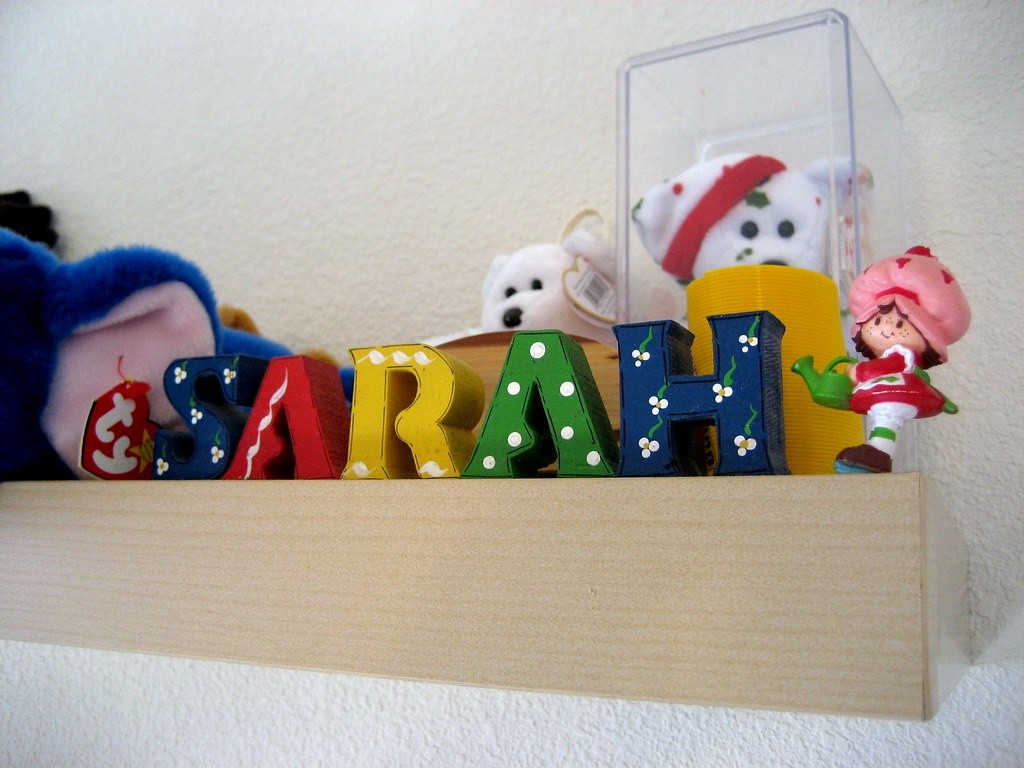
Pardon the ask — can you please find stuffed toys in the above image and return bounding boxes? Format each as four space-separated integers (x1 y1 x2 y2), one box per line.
425 230 617 349
834 246 971 474
1 229 355 480
633 153 875 283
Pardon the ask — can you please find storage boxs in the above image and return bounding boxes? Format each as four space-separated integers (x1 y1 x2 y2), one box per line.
618 6 907 359
